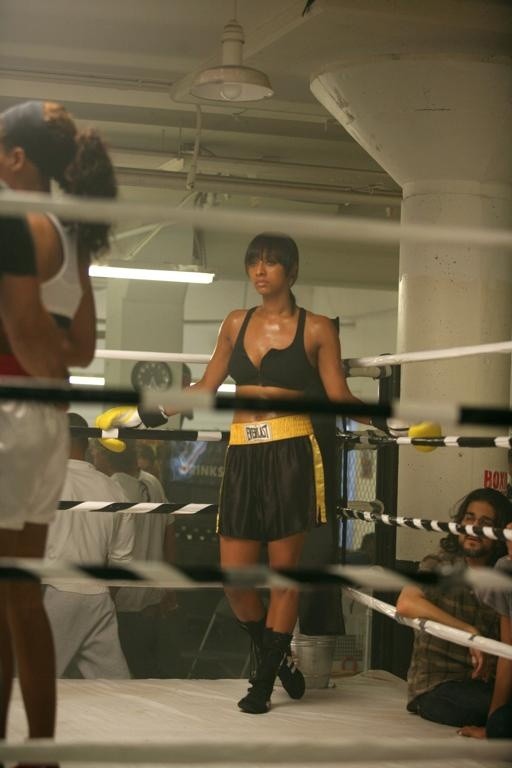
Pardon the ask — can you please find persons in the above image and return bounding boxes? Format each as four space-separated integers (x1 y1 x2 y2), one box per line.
395 487 512 739
0 96 117 768
40 412 131 680
111 231 410 715
90 439 167 679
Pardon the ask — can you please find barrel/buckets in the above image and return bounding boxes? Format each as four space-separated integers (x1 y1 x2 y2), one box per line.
290 634 338 689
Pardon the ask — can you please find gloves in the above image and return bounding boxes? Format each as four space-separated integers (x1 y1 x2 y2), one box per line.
373 415 442 453
95 402 168 452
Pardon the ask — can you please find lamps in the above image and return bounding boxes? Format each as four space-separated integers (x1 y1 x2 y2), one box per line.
189 0 273 103
88 259 215 285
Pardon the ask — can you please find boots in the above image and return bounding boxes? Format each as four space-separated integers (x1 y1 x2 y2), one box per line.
238 628 294 713
238 616 306 700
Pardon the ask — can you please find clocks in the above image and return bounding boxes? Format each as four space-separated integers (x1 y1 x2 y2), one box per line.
131 361 172 393
